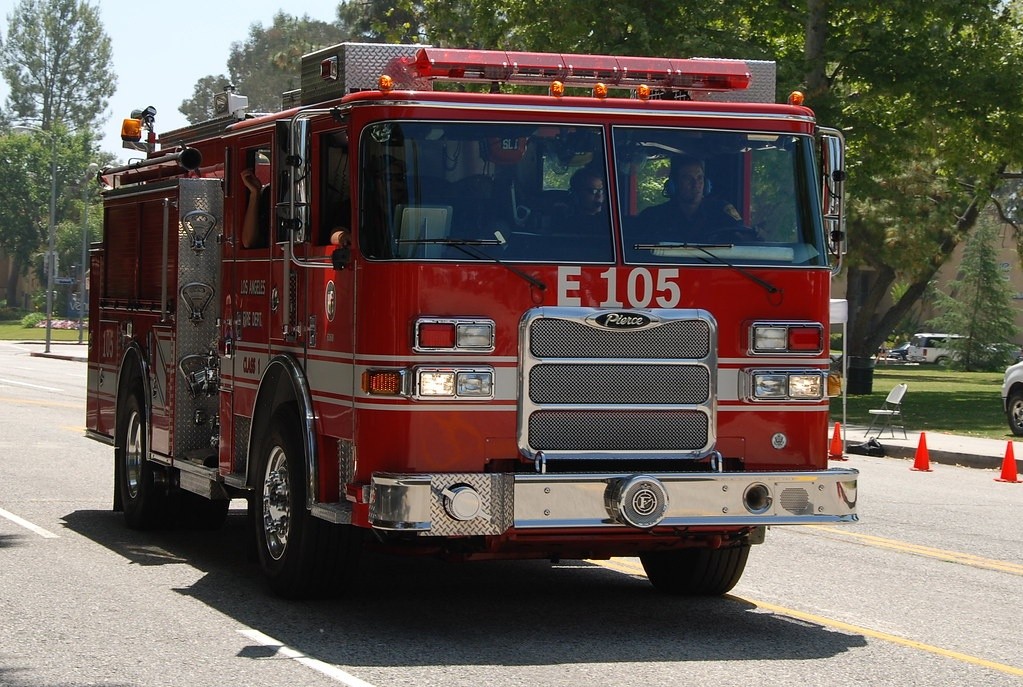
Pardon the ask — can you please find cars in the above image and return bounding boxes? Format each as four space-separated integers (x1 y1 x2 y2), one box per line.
987 342 1022 364
876 341 909 363
1002 360 1023 436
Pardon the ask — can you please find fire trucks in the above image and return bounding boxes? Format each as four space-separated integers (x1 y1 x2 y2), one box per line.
84 36 859 597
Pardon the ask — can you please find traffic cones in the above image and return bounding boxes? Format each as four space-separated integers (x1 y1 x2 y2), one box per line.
911 432 932 473
829 421 849 462
993 440 1023 483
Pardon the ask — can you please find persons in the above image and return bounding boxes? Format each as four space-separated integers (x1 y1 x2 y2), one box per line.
554 168 611 234
240 168 271 248
329 154 410 259
639 154 746 244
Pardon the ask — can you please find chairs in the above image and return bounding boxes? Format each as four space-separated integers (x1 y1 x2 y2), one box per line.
863 383 909 439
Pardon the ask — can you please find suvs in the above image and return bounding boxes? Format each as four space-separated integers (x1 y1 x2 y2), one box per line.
905 333 996 365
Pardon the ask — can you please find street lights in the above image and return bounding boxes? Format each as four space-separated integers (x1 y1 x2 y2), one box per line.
11 126 57 354
79 162 98 342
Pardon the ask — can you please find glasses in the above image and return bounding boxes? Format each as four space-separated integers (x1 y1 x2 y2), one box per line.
580 188 604 195
